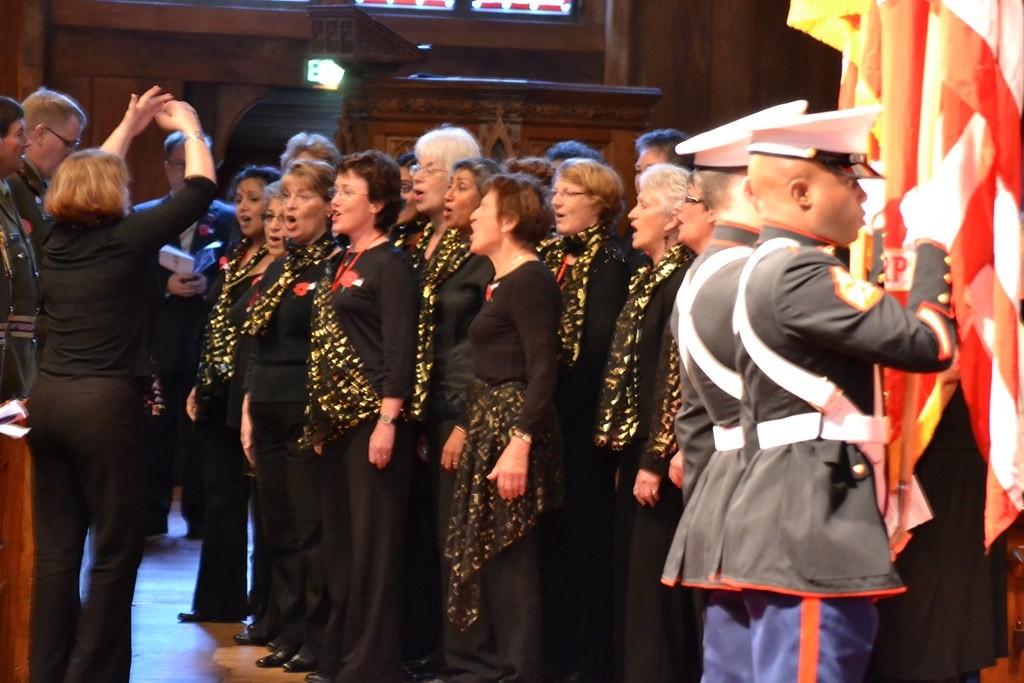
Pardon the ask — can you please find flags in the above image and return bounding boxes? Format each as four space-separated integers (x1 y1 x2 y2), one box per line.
784 0 1023 563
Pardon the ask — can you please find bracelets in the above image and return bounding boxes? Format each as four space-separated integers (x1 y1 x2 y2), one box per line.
508 426 533 444
184 130 213 149
454 424 467 434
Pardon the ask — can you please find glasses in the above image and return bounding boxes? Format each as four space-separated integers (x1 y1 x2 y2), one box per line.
550 189 587 200
328 186 369 200
408 165 449 177
261 213 286 225
166 161 185 171
32 127 83 150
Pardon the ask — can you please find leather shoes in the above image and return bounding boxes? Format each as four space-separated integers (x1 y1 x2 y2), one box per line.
256 653 283 667
282 654 314 672
305 672 333 683
247 623 256 631
267 641 279 650
234 634 256 645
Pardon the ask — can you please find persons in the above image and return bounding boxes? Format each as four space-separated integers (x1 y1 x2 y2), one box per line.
4 88 87 264
0 98 40 403
651 101 959 682
170 119 709 683
125 128 243 542
23 84 216 683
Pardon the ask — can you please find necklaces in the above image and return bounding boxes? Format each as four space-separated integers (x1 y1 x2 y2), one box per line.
499 250 535 276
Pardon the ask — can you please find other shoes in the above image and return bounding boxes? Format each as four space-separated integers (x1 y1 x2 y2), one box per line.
177 609 216 621
189 523 205 538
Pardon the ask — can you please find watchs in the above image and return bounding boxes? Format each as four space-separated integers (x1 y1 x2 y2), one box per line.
377 414 397 426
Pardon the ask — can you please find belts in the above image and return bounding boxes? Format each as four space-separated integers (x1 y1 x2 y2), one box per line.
757 411 890 449
9 316 36 338
713 425 744 452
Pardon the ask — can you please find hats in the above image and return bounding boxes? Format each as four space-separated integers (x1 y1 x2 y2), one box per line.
746 103 884 179
675 99 810 171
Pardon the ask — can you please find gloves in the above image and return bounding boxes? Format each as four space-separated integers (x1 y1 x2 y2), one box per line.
899 181 963 252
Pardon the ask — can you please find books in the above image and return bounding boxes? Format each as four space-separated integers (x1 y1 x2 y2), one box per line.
159 241 223 274
